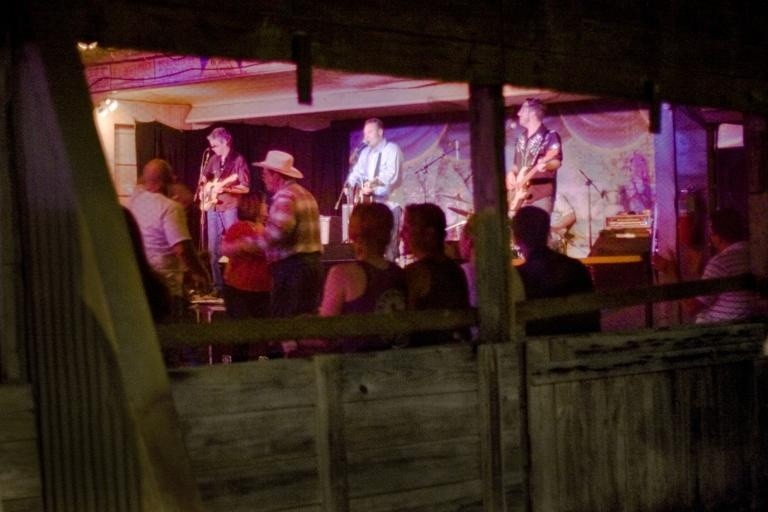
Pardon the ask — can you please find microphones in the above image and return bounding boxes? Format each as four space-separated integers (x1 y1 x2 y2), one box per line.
355 139 367 149
455 139 461 160
207 144 219 150
506 122 516 134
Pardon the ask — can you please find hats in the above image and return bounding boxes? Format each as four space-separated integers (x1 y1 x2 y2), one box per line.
251 150 304 180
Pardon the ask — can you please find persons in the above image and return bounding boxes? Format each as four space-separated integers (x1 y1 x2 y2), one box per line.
223 192 272 364
511 206 602 333
198 128 252 300
255 150 324 319
687 207 752 323
456 215 482 343
509 99 562 214
128 158 197 327
289 202 405 354
398 205 470 347
344 120 404 208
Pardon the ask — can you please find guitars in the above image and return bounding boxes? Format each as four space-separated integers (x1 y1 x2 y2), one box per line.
508 149 559 211
352 177 386 207
199 174 238 211
